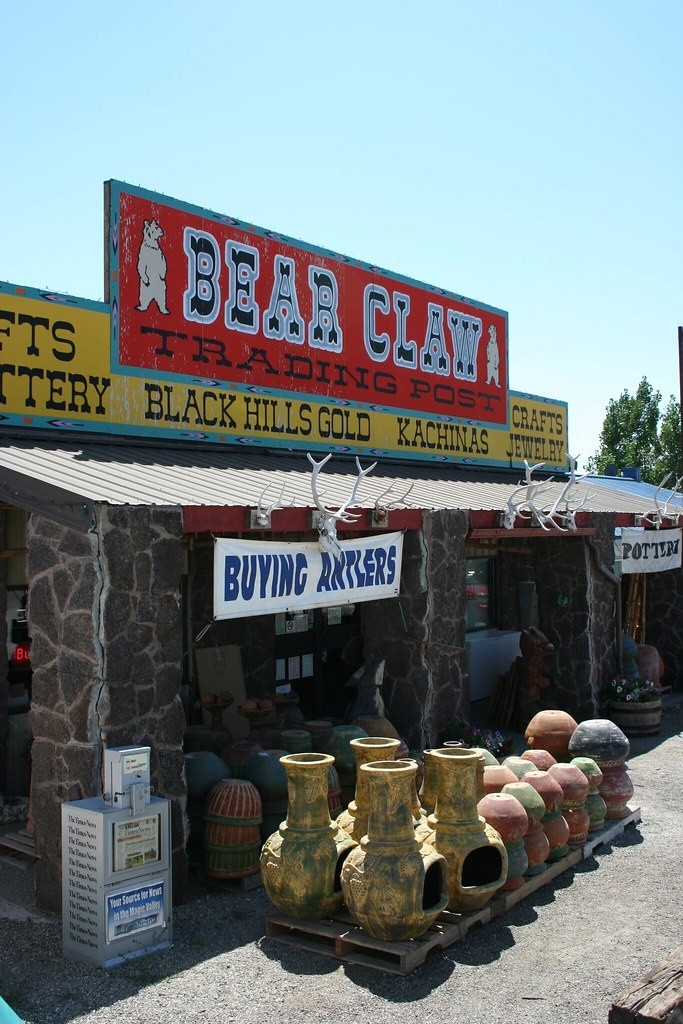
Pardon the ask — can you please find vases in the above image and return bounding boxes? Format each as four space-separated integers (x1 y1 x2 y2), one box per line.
184 706 408 877
328 765 343 820
622 634 665 687
608 700 661 737
477 710 633 890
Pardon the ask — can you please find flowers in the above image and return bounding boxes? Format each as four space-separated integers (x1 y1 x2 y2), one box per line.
438 720 516 757
599 670 660 706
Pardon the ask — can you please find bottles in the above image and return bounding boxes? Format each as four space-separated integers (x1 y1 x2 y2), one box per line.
259 737 509 942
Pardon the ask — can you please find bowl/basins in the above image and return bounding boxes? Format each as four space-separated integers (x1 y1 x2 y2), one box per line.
183 714 410 878
